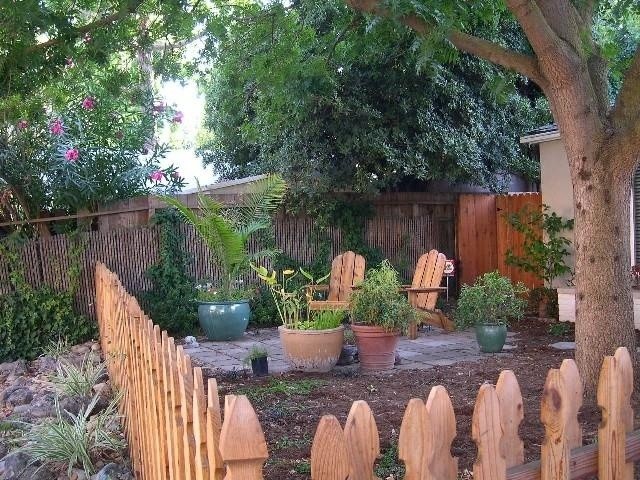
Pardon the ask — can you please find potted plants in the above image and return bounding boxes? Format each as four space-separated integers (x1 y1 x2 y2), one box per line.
455 268 530 352
247 344 269 377
156 170 287 341
251 262 345 373
501 202 575 318
348 259 431 370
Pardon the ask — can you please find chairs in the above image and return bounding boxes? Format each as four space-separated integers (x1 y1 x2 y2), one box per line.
397 249 456 340
306 252 365 323
